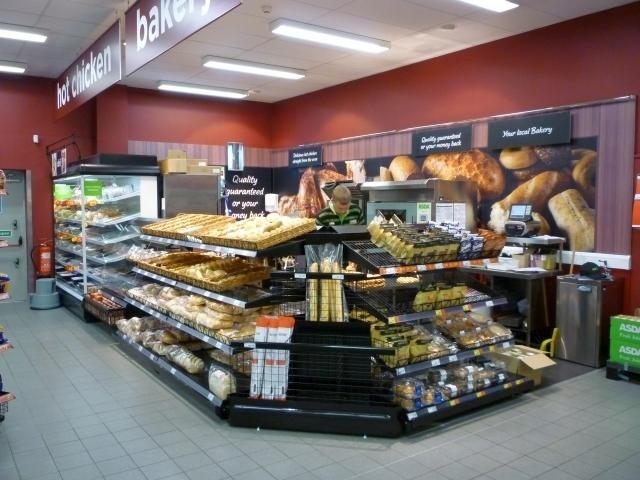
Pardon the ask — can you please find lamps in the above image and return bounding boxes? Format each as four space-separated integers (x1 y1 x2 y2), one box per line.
158 18 391 99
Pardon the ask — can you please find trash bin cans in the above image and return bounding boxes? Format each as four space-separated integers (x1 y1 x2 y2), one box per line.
557 271 624 369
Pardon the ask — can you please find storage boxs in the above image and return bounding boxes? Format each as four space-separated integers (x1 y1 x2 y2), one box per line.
491 344 557 387
159 149 223 176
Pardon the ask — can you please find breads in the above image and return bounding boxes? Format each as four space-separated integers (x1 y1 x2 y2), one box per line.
116 214 281 399
422 151 504 200
490 170 573 232
380 156 425 181
297 159 365 218
548 189 595 252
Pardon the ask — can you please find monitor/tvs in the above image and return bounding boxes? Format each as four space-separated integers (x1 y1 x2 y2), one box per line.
509 205 533 222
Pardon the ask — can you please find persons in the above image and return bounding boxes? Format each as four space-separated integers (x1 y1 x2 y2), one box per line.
315 184 366 227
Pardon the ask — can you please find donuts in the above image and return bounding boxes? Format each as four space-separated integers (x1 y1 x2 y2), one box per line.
500 145 597 208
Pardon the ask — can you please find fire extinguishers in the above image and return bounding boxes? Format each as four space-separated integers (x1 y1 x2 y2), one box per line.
31 240 53 277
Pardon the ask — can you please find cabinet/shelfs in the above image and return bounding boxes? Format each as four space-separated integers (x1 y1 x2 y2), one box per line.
113 212 534 440
52 162 161 323
